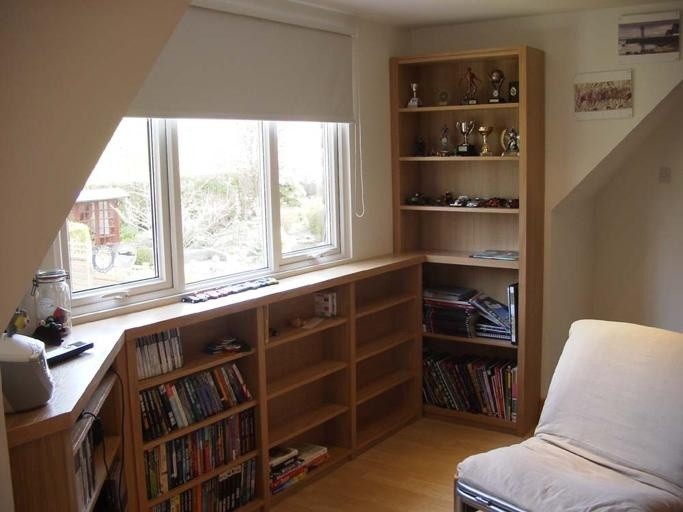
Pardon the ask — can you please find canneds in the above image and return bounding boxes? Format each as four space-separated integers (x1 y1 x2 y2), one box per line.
508 80 519 102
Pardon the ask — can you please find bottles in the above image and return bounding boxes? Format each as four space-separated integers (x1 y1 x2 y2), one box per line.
30 268 73 336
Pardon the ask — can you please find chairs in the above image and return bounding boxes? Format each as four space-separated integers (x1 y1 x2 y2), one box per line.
452 318 681 511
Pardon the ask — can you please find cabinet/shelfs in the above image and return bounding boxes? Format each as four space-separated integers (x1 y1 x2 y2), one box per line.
386 46 544 437
7 317 136 511
125 251 423 512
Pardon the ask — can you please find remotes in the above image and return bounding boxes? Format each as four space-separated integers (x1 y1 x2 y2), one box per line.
47 339 94 363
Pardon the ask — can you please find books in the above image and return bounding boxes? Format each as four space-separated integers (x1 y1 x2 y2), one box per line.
73 427 96 508
134 328 329 512
423 282 518 423
